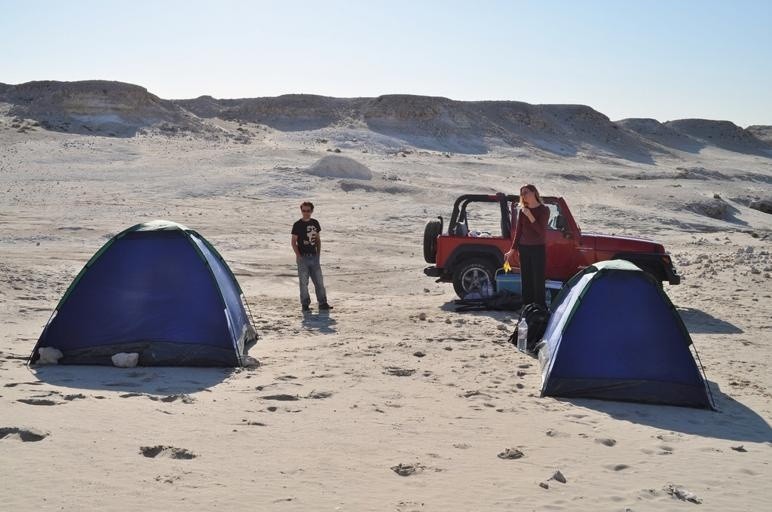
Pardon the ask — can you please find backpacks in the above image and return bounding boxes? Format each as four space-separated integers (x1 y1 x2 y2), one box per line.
510 304 550 354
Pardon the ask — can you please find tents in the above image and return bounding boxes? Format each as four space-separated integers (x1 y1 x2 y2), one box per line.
26 220 260 370
532 258 720 412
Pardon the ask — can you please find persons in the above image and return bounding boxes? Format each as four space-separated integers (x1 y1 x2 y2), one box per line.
503 185 550 310
291 201 334 310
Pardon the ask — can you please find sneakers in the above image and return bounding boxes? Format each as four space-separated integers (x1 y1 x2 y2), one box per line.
319 303 334 309
303 305 314 311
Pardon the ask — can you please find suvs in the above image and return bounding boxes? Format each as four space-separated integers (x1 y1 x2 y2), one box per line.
424 194 680 303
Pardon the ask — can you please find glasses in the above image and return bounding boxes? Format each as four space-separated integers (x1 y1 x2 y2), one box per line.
301 209 310 213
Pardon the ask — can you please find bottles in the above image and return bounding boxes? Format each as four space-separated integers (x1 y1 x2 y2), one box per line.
517 317 528 354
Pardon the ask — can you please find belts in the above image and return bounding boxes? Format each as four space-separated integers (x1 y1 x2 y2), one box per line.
303 253 315 256
